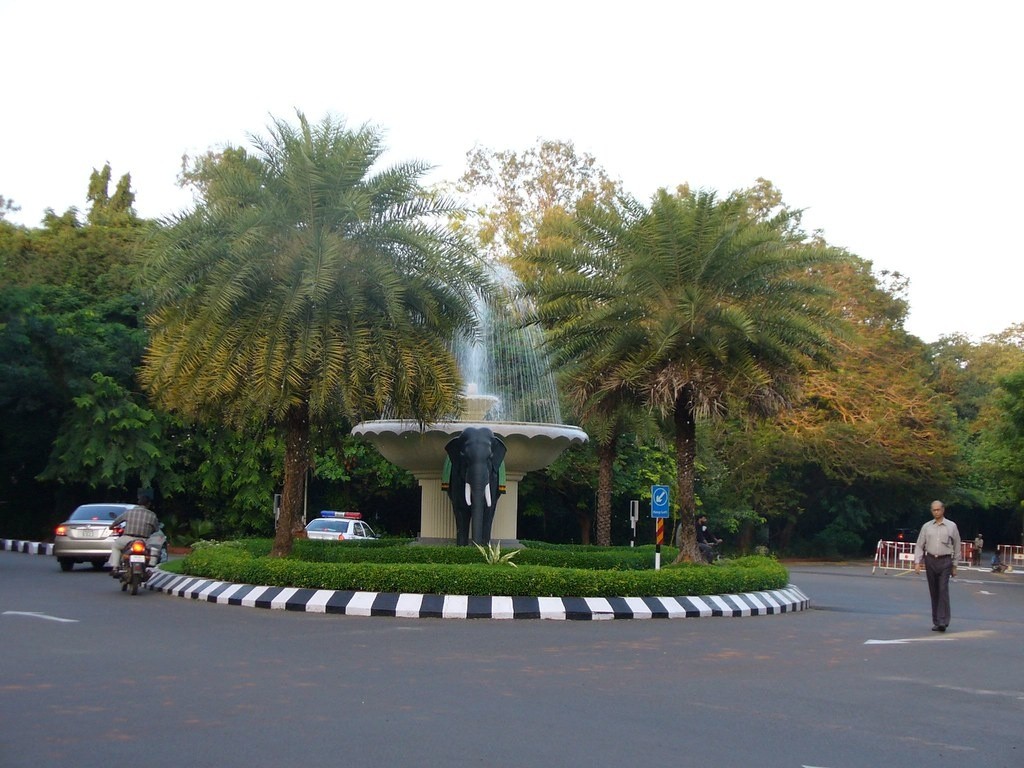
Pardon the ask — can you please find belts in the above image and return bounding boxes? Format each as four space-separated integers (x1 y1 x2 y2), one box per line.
927 553 951 559
124 534 148 538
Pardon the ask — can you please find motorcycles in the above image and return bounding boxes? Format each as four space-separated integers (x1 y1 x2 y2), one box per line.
109 511 165 596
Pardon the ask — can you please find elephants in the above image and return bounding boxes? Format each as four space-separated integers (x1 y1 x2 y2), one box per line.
441 428 506 547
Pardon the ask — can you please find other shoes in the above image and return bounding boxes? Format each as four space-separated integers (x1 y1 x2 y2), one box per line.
932 625 939 631
938 624 947 631
108 569 118 576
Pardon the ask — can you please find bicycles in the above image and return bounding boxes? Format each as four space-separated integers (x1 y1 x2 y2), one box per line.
706 539 725 561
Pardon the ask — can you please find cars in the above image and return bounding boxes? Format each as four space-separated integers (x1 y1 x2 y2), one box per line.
55 502 168 572
306 510 381 542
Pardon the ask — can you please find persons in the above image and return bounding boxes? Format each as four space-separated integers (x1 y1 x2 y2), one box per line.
914 500 961 630
992 550 1008 572
973 534 983 566
676 515 723 563
109 495 159 576
